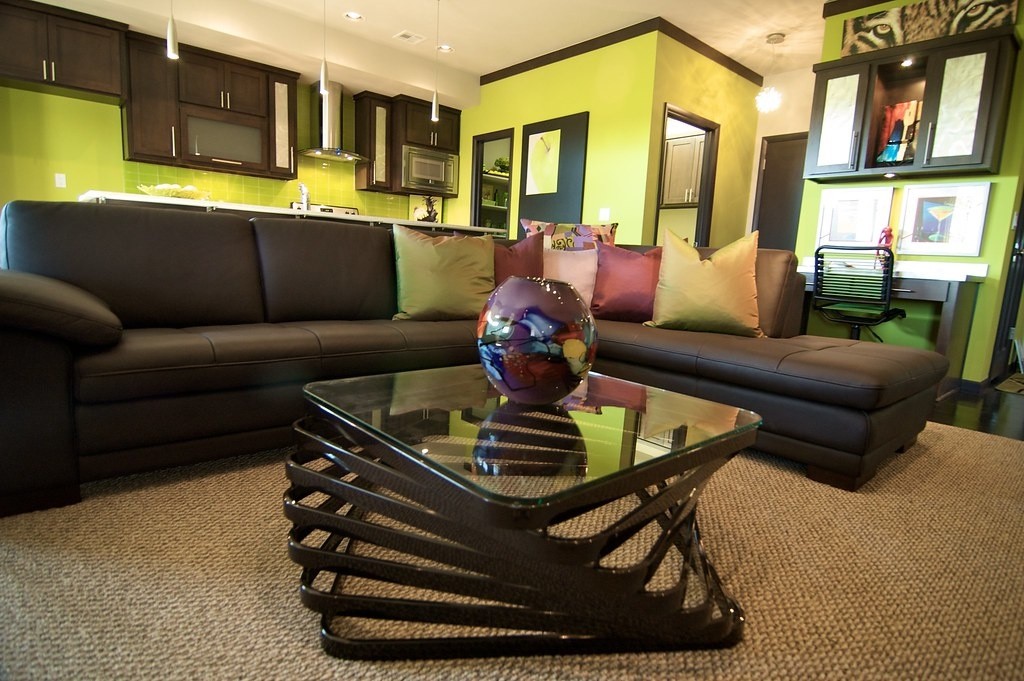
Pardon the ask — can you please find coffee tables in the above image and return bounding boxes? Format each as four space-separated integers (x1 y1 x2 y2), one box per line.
282 361 763 660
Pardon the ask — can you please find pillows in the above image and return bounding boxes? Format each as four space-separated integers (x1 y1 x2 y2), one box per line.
642 230 766 337
393 223 496 321
520 218 619 253
453 230 545 289
540 246 600 309
590 238 690 321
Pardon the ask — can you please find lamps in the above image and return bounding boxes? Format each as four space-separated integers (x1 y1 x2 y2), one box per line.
167 0 180 60
755 34 785 112
319 1 329 95
432 0 442 122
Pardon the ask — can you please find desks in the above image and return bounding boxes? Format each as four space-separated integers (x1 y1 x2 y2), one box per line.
797 271 979 401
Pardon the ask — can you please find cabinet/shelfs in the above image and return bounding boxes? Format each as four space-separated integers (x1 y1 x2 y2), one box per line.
0 0 129 106
802 23 1022 182
352 91 460 197
481 170 509 237
120 29 302 184
657 133 705 209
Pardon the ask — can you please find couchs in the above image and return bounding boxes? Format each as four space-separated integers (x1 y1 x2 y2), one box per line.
0 198 946 518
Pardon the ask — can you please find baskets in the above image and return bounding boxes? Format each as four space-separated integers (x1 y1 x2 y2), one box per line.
137 184 212 199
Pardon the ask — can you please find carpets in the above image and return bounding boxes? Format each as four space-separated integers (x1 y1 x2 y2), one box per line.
1 420 1024 681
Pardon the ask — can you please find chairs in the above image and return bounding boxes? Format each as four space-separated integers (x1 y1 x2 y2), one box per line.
811 245 906 341
